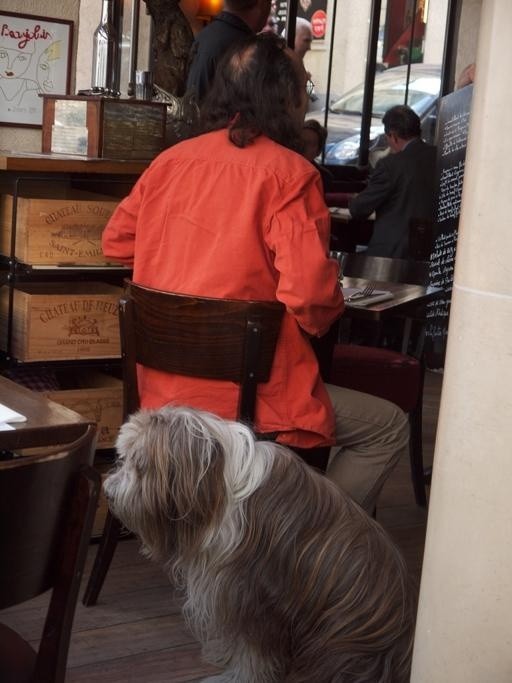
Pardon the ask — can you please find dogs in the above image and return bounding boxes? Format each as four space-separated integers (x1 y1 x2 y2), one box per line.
99 404 416 682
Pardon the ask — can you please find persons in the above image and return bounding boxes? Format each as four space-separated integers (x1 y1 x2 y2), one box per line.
185 0 274 103
295 116 335 193
279 16 315 59
95 25 416 522
347 99 442 289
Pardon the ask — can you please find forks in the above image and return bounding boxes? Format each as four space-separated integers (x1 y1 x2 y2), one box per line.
344 280 377 302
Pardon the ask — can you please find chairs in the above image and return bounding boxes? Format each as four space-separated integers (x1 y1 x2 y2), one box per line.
0 424 100 683
82 252 427 609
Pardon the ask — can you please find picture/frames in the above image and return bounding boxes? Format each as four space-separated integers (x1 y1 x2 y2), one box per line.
0 11 74 129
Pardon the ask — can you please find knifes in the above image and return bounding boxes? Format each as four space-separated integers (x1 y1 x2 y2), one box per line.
347 292 385 303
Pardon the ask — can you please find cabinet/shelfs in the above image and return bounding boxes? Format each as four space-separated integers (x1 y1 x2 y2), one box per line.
0 152 146 544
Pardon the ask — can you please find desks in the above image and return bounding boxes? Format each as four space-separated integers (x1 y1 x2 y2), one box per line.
0 373 85 448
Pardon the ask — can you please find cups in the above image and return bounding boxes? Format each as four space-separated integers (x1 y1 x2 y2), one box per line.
329 251 348 282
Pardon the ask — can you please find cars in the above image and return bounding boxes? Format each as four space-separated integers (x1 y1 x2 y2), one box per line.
304 62 442 166
